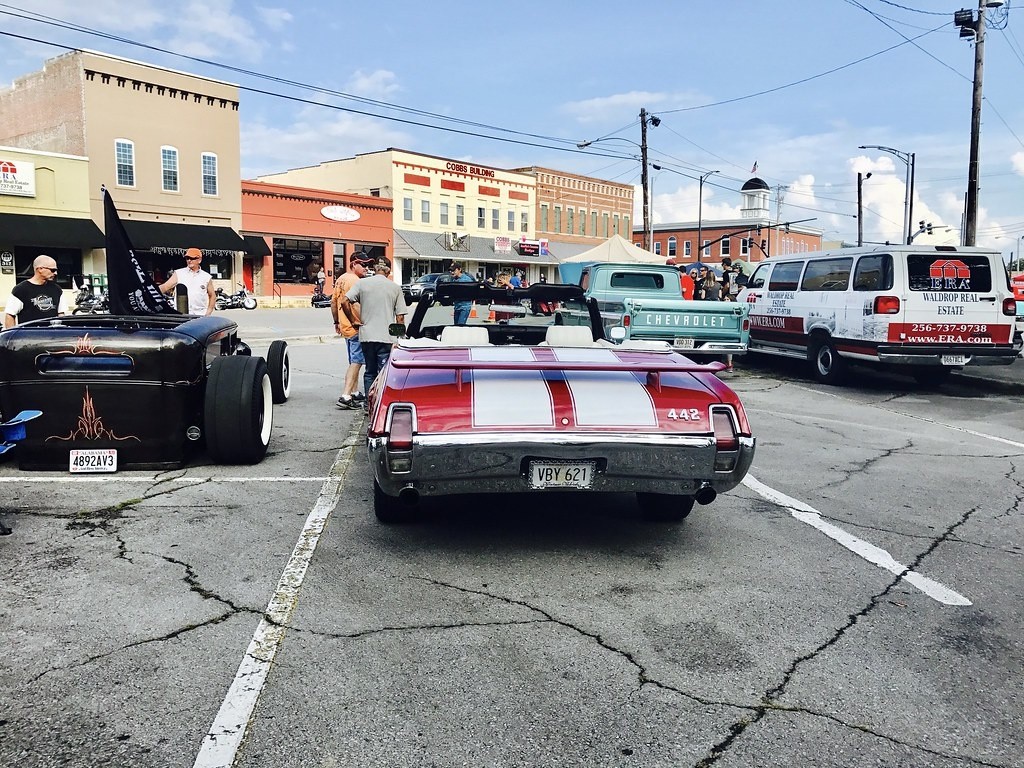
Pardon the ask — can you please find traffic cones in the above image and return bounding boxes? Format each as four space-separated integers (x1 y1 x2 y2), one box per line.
468 300 495 319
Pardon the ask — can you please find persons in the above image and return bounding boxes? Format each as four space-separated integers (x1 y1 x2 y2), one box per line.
4 254 69 329
331 252 375 409
159 248 217 316
316 266 326 293
448 262 549 325
340 256 408 415
667 257 750 373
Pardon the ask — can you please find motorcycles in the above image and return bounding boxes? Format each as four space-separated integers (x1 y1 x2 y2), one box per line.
311 284 335 308
72 284 109 314
214 280 258 311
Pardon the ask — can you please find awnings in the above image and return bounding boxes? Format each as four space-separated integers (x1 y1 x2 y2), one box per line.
0 213 108 248
120 220 248 256
242 235 272 257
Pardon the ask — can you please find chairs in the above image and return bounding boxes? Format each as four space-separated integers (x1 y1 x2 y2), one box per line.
439 325 490 346
540 325 595 346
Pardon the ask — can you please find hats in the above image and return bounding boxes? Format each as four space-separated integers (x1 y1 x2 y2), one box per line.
449 262 461 270
350 252 375 265
183 248 203 258
375 256 391 274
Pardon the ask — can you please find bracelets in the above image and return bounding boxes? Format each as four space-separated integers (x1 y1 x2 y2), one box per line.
334 321 339 324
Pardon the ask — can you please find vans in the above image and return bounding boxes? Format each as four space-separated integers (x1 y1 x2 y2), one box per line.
734 244 1024 385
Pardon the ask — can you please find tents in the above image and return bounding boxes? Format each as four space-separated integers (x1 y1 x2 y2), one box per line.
559 234 675 285
685 262 724 281
722 258 757 277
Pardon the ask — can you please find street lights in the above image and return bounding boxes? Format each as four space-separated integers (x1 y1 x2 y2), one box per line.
859 145 915 245
577 137 650 251
995 236 1020 276
698 170 720 262
945 229 962 246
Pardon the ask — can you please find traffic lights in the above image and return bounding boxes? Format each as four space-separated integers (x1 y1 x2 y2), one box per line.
761 239 766 249
919 221 925 233
785 222 790 235
927 223 933 234
749 237 754 247
757 224 762 236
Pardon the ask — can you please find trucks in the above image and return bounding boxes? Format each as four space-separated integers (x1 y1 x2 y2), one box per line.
553 262 752 364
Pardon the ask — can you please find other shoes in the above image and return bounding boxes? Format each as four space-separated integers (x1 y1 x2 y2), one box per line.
364 405 369 414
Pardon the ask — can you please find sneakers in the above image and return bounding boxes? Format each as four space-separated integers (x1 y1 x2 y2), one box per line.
350 392 365 403
336 397 362 410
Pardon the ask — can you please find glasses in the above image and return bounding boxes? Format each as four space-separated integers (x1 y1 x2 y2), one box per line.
692 272 697 275
356 261 371 268
36 267 58 273
700 270 706 272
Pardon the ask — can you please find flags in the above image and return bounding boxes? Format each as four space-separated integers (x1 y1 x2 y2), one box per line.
751 161 758 173
101 184 182 316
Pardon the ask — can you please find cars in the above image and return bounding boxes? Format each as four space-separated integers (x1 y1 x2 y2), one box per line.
401 272 475 308
0 313 292 474
366 281 758 525
